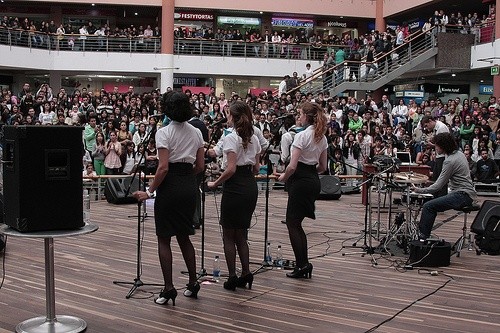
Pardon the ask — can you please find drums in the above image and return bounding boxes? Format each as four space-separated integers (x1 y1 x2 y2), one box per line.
401 194 432 208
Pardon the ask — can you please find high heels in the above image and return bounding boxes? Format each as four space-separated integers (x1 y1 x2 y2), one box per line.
224 275 238 291
238 273 254 289
153 289 178 307
182 282 201 299
286 263 314 279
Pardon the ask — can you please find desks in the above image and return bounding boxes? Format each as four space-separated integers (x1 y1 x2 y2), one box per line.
0 226 101 333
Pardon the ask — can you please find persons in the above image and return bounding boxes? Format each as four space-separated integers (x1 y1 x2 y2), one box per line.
201 101 262 291
132 90 205 306
0 3 500 199
186 102 209 228
411 132 477 241
277 102 327 280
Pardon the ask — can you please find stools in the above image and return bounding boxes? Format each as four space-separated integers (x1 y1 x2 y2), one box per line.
453 207 483 257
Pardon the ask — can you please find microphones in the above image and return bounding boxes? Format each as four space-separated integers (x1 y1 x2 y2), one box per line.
149 114 165 119
392 158 399 169
277 114 294 120
213 118 227 126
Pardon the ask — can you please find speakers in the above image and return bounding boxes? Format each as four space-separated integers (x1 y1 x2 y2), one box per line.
471 200 500 233
103 176 144 204
2 125 86 233
316 175 341 200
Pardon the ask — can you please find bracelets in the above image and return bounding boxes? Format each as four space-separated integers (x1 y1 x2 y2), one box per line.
145 186 154 198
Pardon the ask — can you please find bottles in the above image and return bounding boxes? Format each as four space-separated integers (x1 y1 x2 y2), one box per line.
83 190 91 226
266 243 272 265
276 245 282 270
212 256 221 283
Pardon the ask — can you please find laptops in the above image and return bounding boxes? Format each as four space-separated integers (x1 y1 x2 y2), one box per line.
396 152 418 165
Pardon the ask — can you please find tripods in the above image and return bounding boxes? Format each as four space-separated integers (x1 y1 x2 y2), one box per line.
249 119 295 276
338 160 419 266
180 123 229 286
112 119 165 298
